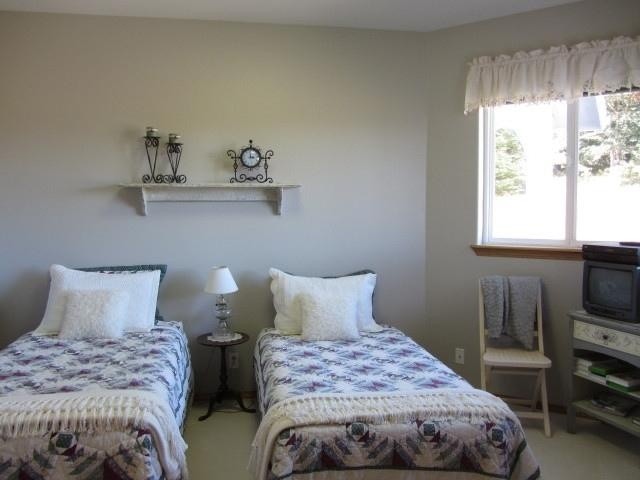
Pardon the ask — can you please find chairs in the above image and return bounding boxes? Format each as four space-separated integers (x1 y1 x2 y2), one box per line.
478 276 551 438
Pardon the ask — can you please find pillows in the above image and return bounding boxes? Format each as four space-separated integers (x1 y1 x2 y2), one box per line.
30 263 167 340
267 267 385 342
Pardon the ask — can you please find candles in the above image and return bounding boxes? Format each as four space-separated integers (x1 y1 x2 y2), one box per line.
168 133 181 144
145 126 160 137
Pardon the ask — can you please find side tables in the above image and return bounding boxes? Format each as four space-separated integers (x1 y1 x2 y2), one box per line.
197 332 256 421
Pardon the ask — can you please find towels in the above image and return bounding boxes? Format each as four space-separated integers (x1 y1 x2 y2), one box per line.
481 276 539 349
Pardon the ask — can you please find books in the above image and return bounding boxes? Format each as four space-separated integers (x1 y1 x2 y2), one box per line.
592 399 640 427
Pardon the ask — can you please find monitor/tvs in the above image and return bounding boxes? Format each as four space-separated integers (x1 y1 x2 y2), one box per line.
582 262 640 323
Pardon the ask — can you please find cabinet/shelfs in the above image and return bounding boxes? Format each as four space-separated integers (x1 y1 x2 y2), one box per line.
566 311 640 438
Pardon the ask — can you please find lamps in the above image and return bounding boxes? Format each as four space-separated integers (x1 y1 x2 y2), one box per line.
204 266 243 342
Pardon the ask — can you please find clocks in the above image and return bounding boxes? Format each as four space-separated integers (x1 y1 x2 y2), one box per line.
227 139 274 183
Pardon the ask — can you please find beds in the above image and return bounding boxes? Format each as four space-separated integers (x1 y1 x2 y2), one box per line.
0 322 196 480
245 324 540 480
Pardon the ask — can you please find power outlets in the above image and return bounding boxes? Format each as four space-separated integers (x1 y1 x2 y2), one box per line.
455 348 465 364
229 352 240 368
